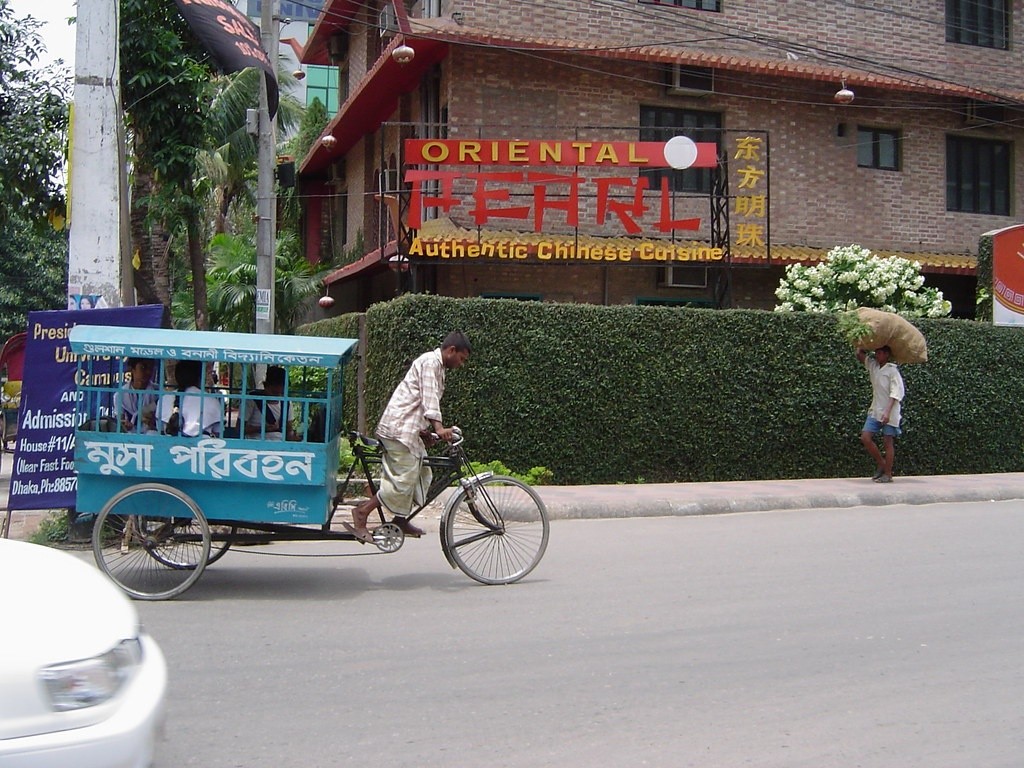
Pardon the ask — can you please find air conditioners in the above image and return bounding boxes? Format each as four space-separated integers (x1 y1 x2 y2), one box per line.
657 266 706 289
377 167 397 196
665 63 714 98
379 5 400 38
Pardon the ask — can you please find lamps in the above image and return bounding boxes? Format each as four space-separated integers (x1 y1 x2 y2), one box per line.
320 133 337 152
392 36 415 63
389 253 409 273
834 80 855 104
318 284 336 308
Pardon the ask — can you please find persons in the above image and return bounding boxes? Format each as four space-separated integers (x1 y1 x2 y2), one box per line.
857 345 906 483
113 356 171 434
342 331 473 542
236 367 300 442
156 360 223 437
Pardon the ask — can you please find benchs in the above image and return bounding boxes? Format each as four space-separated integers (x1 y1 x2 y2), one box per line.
0 382 23 408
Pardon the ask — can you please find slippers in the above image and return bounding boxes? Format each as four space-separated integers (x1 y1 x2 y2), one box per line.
387 526 425 534
872 466 883 480
343 522 374 543
876 473 895 483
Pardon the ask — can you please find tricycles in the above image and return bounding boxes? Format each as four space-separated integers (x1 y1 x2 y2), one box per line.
68 324 551 602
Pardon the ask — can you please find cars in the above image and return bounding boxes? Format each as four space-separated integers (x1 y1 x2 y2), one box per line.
1 537 169 767
